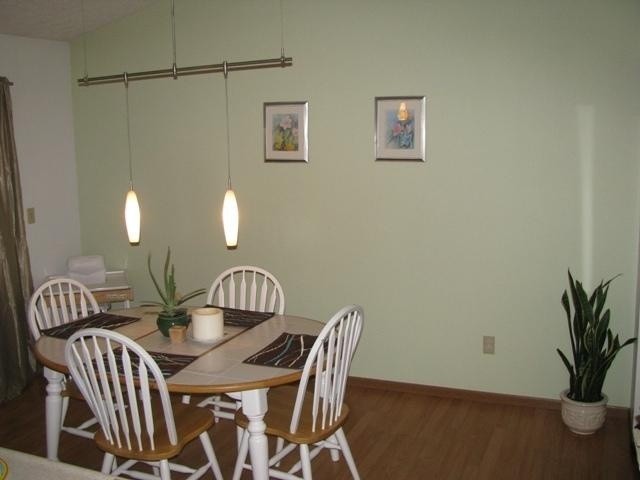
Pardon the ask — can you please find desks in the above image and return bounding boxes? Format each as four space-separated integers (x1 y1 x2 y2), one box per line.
34 306 341 480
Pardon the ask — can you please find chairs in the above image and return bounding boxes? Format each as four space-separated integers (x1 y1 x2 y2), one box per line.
182 264 288 424
61 327 227 479
234 302 365 479
25 277 129 445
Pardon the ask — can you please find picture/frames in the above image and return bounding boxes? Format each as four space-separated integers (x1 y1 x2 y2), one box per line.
374 95 427 162
262 100 309 163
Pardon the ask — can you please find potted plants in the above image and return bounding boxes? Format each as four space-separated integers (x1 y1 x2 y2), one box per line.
557 265 637 437
138 245 209 335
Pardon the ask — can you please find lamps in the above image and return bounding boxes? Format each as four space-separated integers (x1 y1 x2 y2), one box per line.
72 2 291 250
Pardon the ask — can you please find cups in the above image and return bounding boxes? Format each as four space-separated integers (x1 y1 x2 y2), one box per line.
168 325 188 343
190 307 224 342
